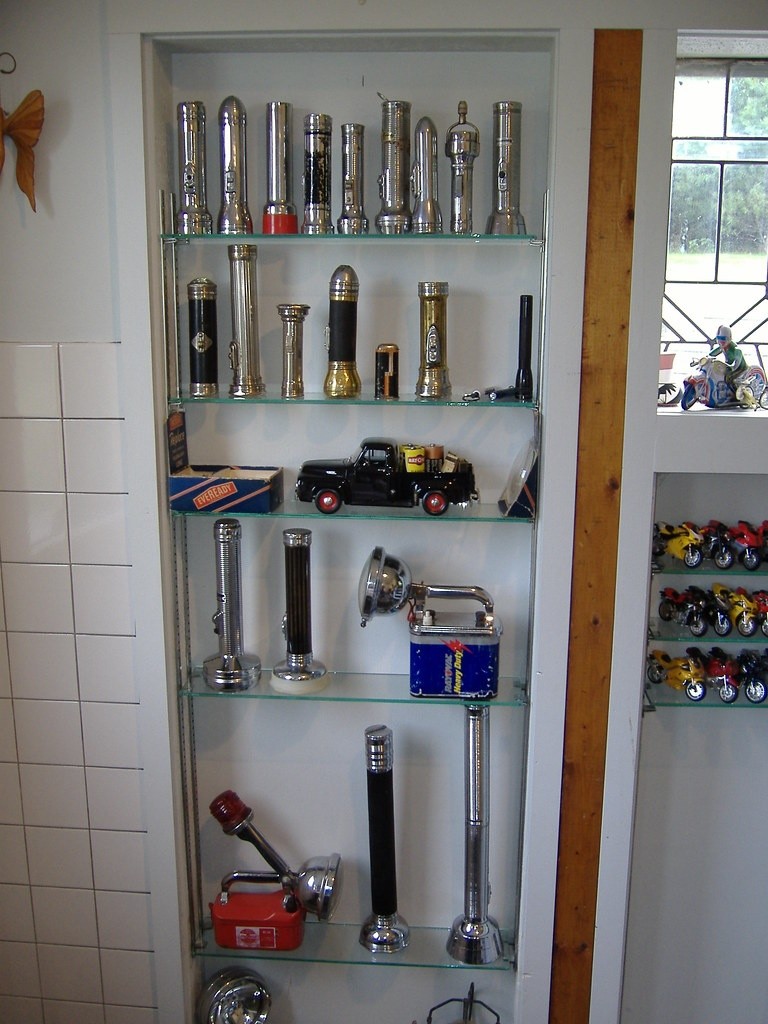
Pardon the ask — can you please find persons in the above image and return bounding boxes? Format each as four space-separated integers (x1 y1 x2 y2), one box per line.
688 325 746 402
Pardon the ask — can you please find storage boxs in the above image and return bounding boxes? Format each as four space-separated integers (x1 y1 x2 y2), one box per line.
169 465 284 513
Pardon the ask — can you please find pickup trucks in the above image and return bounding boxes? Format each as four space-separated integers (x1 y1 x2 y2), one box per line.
292 436 478 512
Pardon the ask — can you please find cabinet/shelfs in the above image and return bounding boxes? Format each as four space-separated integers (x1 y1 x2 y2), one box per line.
110 0 596 1024
641 472 768 712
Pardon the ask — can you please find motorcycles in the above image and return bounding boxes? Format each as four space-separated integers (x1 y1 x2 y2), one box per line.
758 521 768 559
730 520 761 570
707 584 735 637
658 584 711 637
735 648 768 703
704 646 741 704
751 591 768 637
680 357 765 413
728 586 758 637
700 518 734 570
656 517 706 567
646 647 707 701
